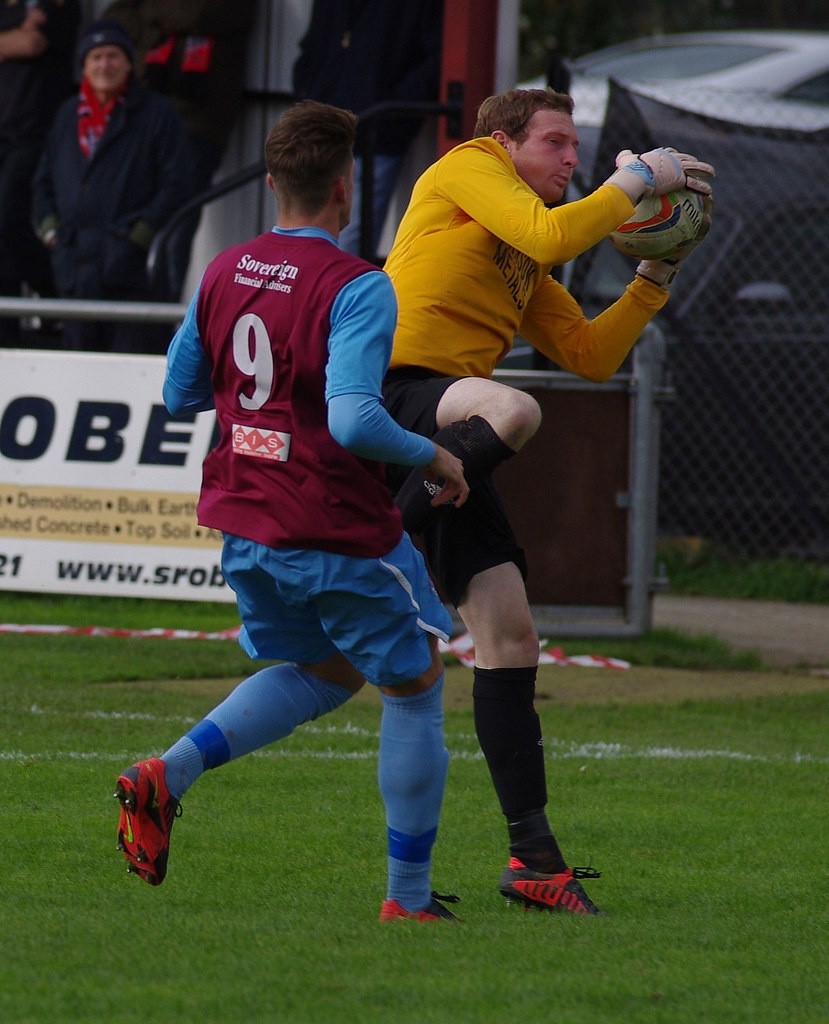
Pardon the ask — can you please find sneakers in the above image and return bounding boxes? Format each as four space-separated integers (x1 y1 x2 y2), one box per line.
112 759 183 886
381 891 465 924
498 857 603 915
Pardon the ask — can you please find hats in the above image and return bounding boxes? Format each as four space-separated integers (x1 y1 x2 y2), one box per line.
77 19 133 71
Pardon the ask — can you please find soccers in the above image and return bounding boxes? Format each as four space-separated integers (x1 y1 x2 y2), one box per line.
606 169 704 260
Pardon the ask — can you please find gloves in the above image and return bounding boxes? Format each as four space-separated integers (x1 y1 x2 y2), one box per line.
636 194 714 291
608 148 715 200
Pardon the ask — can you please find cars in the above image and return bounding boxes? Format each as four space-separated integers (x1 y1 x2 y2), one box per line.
373 23 828 569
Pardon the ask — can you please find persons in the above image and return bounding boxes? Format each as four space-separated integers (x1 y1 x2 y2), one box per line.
378 89 718 915
112 102 471 925
0 0 445 356
